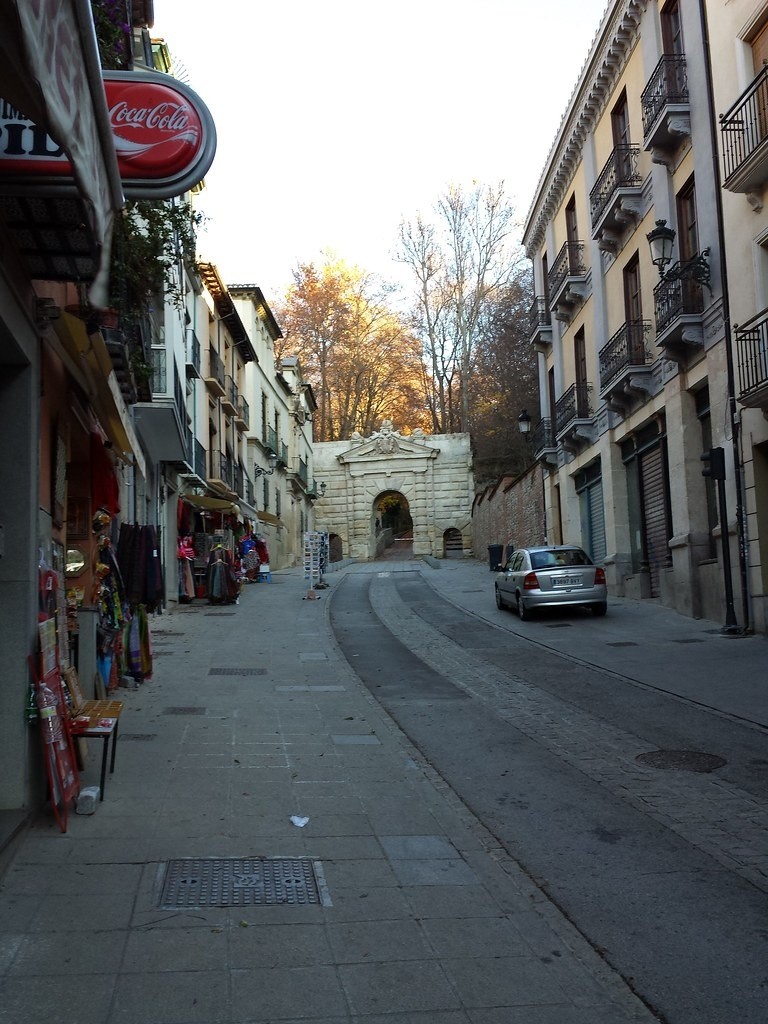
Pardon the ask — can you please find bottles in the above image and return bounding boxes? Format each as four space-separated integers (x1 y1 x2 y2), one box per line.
24 684 37 726
36 683 62 744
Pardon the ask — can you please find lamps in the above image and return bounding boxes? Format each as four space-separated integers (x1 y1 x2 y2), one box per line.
517 409 537 449
254 452 278 481
646 220 714 298
317 482 326 497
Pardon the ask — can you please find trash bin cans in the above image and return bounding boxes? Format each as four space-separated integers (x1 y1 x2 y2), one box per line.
489 543 504 571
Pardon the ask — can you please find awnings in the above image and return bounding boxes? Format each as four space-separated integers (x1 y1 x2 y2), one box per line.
177 492 285 531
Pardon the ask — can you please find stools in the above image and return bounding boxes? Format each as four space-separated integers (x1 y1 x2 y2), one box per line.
260 572 271 584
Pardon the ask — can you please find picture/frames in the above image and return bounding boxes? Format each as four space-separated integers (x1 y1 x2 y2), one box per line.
66 496 90 540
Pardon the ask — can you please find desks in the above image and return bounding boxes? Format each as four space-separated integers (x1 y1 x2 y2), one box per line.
72 698 124 801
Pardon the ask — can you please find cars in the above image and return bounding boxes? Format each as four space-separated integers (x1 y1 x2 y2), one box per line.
495 545 608 621
